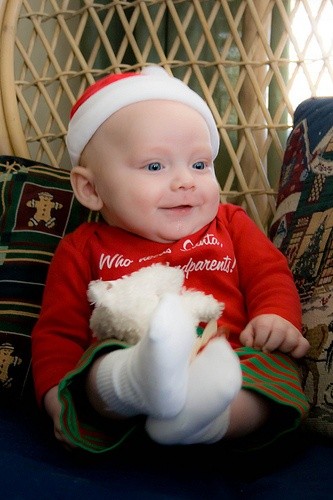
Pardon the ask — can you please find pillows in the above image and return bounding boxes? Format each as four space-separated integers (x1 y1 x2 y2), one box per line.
0 155 106 403
267 98 333 437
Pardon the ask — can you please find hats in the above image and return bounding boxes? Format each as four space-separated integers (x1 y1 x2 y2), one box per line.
64 65 220 170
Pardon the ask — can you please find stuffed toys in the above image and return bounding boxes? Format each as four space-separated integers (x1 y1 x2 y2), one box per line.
86 263 225 346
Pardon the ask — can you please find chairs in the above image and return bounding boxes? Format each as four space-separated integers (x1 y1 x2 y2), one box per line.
0 0 333 500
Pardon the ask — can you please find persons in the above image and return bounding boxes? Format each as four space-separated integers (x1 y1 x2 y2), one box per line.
31 65 311 454
